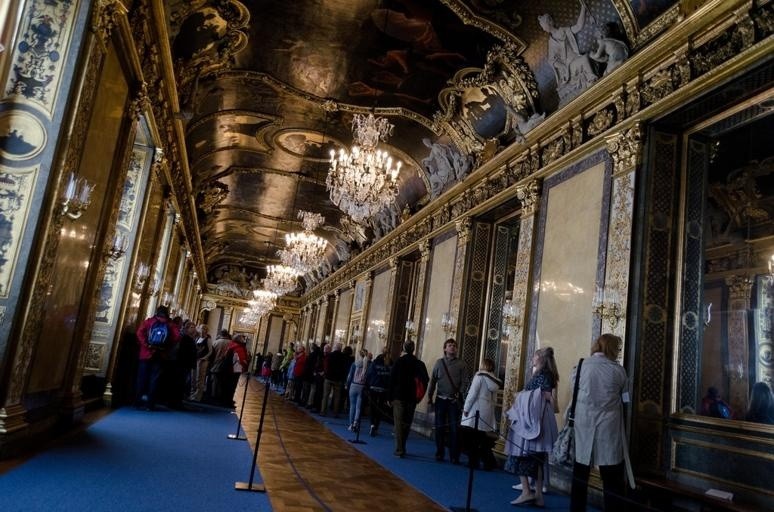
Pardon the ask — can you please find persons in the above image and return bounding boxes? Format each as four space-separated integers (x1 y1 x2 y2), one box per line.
426 338 469 465
385 340 429 459
502 347 560 508
744 380 773 426
129 304 251 411
565 334 630 511
458 357 503 474
587 20 630 75
416 136 458 199
449 151 475 182
534 3 602 110
701 384 736 421
508 356 559 496
257 337 393 436
466 88 504 120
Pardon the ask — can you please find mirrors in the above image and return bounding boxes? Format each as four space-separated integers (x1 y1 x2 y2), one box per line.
662 84 774 435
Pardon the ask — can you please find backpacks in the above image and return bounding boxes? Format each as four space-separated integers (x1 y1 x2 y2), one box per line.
147 316 171 346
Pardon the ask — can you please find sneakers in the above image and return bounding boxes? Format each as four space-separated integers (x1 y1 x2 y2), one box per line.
510 484 548 507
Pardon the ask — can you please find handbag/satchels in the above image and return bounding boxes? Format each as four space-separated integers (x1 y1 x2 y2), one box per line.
455 392 463 409
233 353 243 373
551 425 575 467
415 378 425 402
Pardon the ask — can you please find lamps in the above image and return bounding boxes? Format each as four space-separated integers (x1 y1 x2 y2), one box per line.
501 302 522 336
441 312 456 336
103 233 129 261
53 171 96 221
591 284 625 334
326 110 401 226
404 320 417 337
239 210 328 326
371 319 387 340
